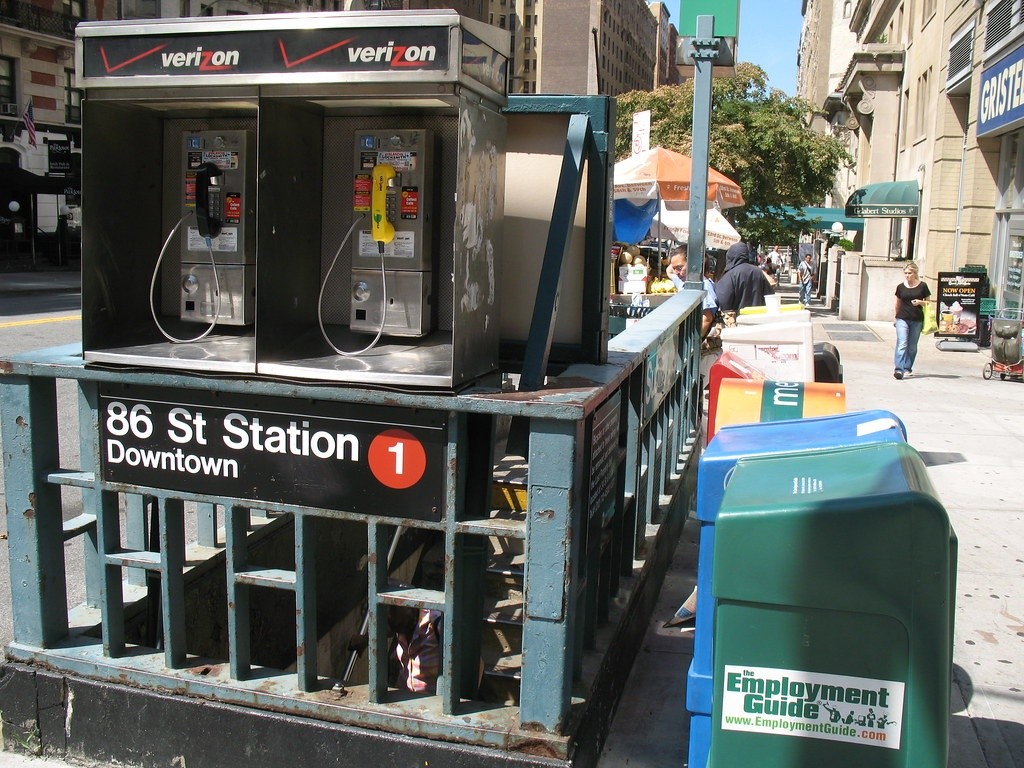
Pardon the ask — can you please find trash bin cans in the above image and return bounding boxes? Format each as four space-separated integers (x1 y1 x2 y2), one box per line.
713 444 958 768
687 409 911 768
814 342 843 383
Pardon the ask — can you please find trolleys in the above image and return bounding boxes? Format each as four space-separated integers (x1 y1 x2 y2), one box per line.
981 311 1024 380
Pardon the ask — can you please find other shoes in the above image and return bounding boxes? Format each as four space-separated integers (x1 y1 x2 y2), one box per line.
799 300 803 305
893 371 904 380
904 371 914 376
806 303 809 307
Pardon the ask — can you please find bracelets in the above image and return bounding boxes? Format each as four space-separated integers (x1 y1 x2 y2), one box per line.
698 333 703 341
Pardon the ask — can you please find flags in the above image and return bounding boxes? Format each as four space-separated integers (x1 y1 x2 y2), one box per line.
23 98 37 149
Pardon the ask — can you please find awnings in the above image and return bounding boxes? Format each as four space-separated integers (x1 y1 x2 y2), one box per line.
733 181 919 230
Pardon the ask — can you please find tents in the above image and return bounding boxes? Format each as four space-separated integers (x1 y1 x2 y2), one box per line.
0 162 64 265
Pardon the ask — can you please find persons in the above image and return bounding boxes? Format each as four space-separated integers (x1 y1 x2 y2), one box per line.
8 213 21 240
893 260 932 379
615 244 787 343
54 215 69 266
796 254 817 306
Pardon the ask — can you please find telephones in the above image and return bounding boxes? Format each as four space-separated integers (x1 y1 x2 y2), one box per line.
370 166 394 245
197 162 219 240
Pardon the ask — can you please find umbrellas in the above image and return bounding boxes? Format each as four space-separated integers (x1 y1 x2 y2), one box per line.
613 146 741 281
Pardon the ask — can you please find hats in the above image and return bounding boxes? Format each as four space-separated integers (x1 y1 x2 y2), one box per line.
767 257 772 263
